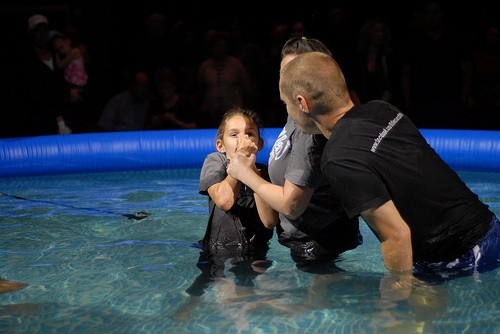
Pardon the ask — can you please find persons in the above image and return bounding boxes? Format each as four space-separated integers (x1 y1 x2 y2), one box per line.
279 51 499 303
225 35 364 276
167 106 281 326
25 13 245 130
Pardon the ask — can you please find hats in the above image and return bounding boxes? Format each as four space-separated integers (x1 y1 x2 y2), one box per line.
28 14 48 29
